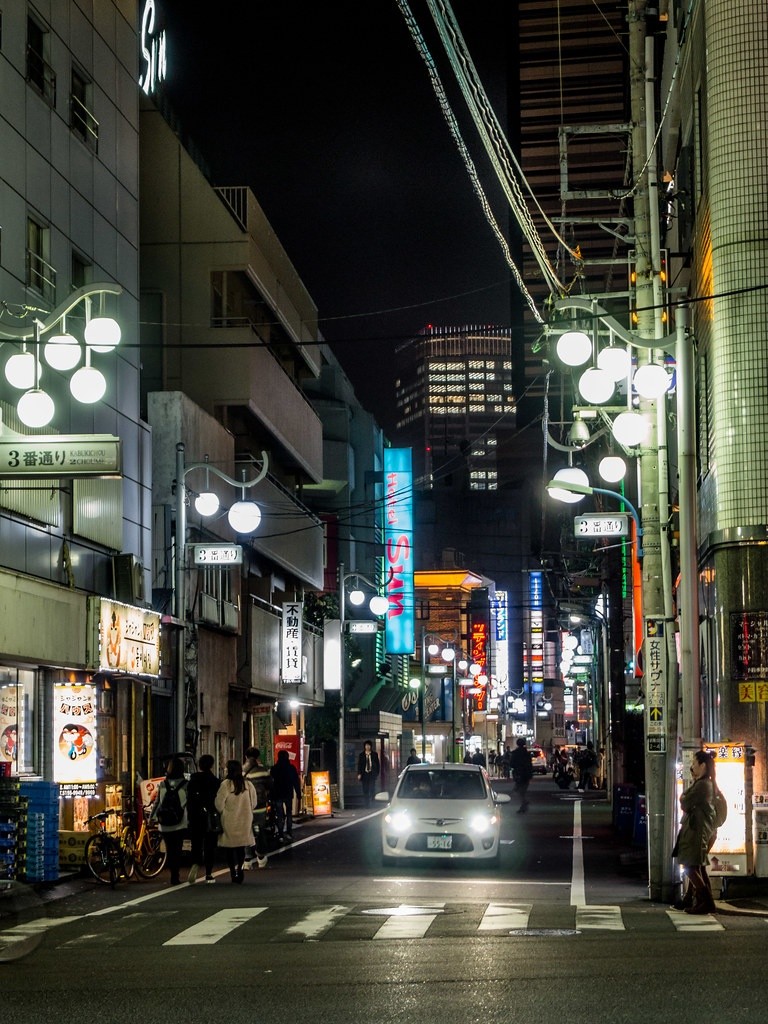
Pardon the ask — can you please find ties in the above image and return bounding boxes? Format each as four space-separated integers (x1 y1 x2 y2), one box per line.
367 754 370 772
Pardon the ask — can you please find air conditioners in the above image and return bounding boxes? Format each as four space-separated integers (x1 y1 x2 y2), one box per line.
115 553 146 607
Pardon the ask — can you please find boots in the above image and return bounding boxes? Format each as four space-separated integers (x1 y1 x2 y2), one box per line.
670 893 693 911
684 885 717 915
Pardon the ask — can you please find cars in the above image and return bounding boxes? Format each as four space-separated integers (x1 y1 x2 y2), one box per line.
527 748 547 776
374 762 512 869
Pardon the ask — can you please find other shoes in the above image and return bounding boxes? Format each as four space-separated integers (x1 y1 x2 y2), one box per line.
187 864 199 883
515 802 529 814
286 833 295 840
279 837 284 842
256 855 268 869
232 874 243 886
171 880 183 886
242 857 258 870
204 875 217 885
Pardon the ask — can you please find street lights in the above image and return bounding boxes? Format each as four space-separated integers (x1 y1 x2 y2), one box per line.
503 685 524 750
421 625 458 762
463 672 489 758
486 675 507 773
560 661 595 746
338 563 393 810
176 442 269 761
554 296 702 893
533 692 553 740
558 602 611 804
452 650 484 762
560 649 597 754
559 622 601 748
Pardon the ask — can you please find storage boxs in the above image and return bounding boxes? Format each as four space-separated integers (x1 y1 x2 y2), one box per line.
0 761 97 888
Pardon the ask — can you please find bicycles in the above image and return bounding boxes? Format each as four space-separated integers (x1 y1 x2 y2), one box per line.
82 809 123 888
119 796 169 881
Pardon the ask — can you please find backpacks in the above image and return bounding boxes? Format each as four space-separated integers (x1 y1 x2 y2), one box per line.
151 776 189 827
702 787 728 827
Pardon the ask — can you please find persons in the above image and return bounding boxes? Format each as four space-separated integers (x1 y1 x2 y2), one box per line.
357 741 380 809
509 737 532 814
148 759 189 886
215 760 257 884
241 747 274 870
473 748 486 766
269 750 302 842
553 741 605 790
670 751 718 915
488 748 511 778
185 754 223 884
407 748 421 765
463 751 473 763
